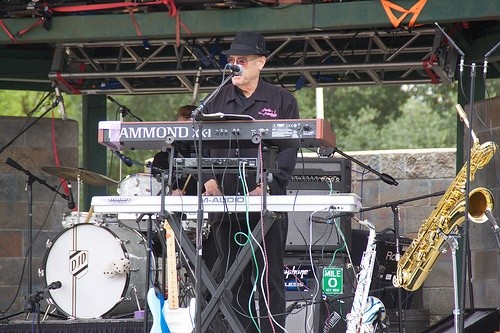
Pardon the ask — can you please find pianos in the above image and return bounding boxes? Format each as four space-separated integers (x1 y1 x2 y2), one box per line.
96 117 339 333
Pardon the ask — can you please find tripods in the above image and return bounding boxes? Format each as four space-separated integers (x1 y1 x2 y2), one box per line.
0 157 68 322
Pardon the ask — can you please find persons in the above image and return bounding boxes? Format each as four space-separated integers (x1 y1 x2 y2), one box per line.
151 105 206 257
190 30 300 333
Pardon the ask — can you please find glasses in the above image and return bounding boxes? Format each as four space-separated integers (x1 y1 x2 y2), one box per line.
227 55 260 65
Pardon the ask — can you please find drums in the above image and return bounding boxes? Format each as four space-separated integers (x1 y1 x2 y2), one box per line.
42 221 149 317
179 201 211 242
118 173 168 232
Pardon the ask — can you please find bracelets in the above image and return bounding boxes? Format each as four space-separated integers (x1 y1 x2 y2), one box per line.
257 183 270 194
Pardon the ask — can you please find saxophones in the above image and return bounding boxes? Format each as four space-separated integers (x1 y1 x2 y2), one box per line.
346 220 387 333
392 141 498 292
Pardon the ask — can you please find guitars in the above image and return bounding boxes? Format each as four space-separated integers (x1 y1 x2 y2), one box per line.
146 218 196 333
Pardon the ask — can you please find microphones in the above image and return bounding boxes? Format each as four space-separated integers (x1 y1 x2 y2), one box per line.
113 150 132 167
484 210 500 232
48 281 62 289
66 180 75 209
52 80 67 120
227 63 243 75
309 216 334 225
284 301 298 318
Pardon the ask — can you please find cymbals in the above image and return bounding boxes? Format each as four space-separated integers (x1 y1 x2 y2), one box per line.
41 163 120 187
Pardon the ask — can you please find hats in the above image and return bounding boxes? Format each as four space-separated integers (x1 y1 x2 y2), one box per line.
222 31 270 57
